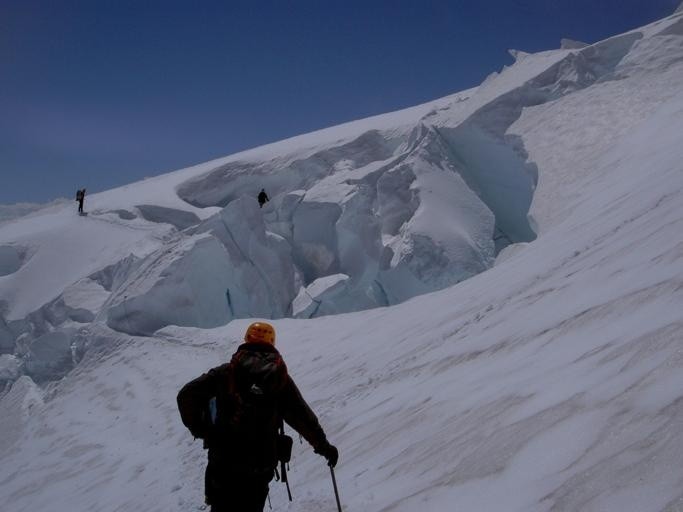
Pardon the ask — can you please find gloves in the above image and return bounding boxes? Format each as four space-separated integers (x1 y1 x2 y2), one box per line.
323 444 338 468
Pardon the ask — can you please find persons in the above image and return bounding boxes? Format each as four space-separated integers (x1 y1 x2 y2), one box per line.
257 188 269 208
176 322 339 512
78 189 86 213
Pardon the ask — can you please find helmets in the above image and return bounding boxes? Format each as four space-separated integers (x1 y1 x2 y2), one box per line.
244 322 275 346
